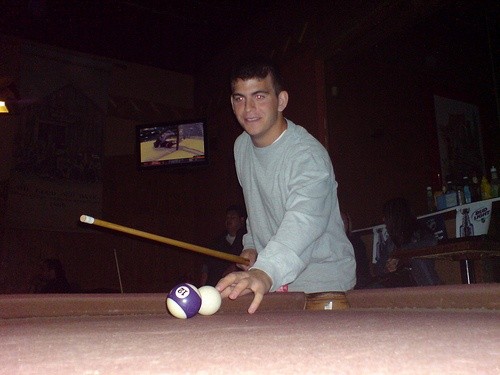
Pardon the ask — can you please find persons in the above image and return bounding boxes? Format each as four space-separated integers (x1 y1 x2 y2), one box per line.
373 199 446 288
340 207 369 287
200 201 252 289
215 59 357 314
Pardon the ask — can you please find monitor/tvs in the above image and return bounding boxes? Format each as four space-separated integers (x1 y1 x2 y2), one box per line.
136 117 210 170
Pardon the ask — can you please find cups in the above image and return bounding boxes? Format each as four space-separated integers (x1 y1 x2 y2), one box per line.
460 258 476 284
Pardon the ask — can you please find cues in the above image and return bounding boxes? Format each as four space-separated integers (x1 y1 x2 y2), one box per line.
80 214 250 266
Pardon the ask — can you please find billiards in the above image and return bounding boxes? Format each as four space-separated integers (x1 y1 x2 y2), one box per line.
197 286 222 315
166 282 202 319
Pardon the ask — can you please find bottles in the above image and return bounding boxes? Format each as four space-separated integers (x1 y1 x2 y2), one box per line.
424 164 499 212
459 208 475 237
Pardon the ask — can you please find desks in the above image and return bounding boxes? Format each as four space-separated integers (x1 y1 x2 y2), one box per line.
406 233 500 283
0 282 500 375
